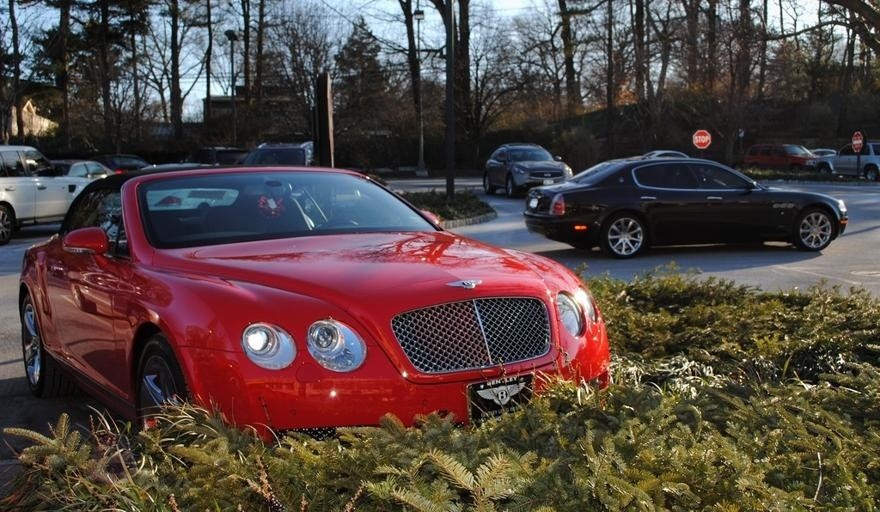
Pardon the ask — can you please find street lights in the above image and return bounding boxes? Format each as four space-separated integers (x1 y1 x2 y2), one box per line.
224 30 239 146
414 0 428 178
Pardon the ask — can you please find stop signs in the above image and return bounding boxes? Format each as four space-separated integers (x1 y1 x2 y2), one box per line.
851 132 863 152
693 130 711 148
